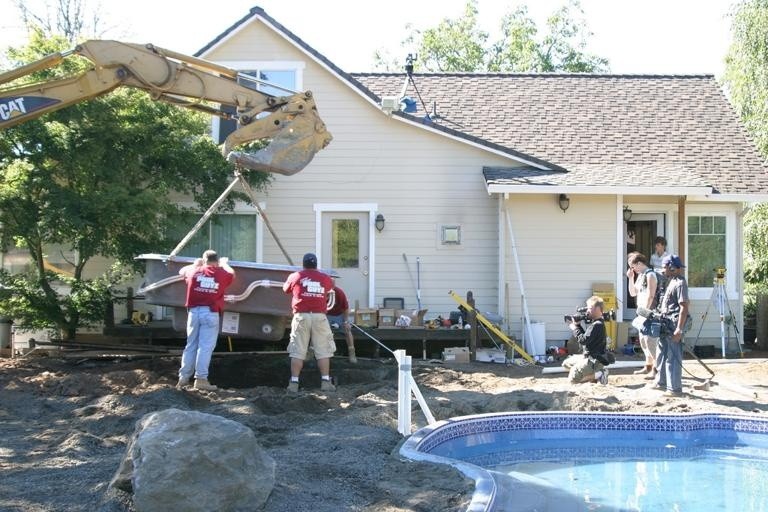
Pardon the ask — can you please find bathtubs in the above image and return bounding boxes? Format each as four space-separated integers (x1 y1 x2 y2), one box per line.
133 250 342 342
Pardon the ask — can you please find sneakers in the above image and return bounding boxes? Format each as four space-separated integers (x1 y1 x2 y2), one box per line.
347 349 357 364
634 369 649 374
286 382 299 394
644 374 655 380
600 369 608 384
321 381 337 392
177 379 190 387
193 378 217 391
662 390 682 397
651 385 667 390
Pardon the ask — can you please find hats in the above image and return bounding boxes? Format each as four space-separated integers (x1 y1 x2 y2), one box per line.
303 253 317 266
663 254 685 268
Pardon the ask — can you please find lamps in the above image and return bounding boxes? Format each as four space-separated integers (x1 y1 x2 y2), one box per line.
559 194 570 212
375 213 386 233
623 204 632 222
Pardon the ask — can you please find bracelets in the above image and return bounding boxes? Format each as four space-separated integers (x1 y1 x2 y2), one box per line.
343 320 349 323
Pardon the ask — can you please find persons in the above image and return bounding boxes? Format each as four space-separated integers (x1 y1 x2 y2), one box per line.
626 252 661 380
177 250 236 390
652 254 689 397
562 295 609 387
326 288 357 363
650 236 672 275
283 253 337 393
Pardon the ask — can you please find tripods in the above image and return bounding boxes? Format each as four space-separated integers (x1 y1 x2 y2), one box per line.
400 75 431 121
691 278 745 358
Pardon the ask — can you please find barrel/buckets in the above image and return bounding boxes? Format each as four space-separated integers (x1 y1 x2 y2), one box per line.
523 319 547 356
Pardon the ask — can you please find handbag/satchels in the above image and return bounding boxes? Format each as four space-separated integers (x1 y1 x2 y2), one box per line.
596 352 616 366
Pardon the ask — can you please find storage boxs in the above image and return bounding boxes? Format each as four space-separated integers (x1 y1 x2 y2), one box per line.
442 346 470 363
475 348 507 364
347 307 428 326
592 282 628 350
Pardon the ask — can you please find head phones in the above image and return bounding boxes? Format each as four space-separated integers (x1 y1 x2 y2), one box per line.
668 254 675 271
